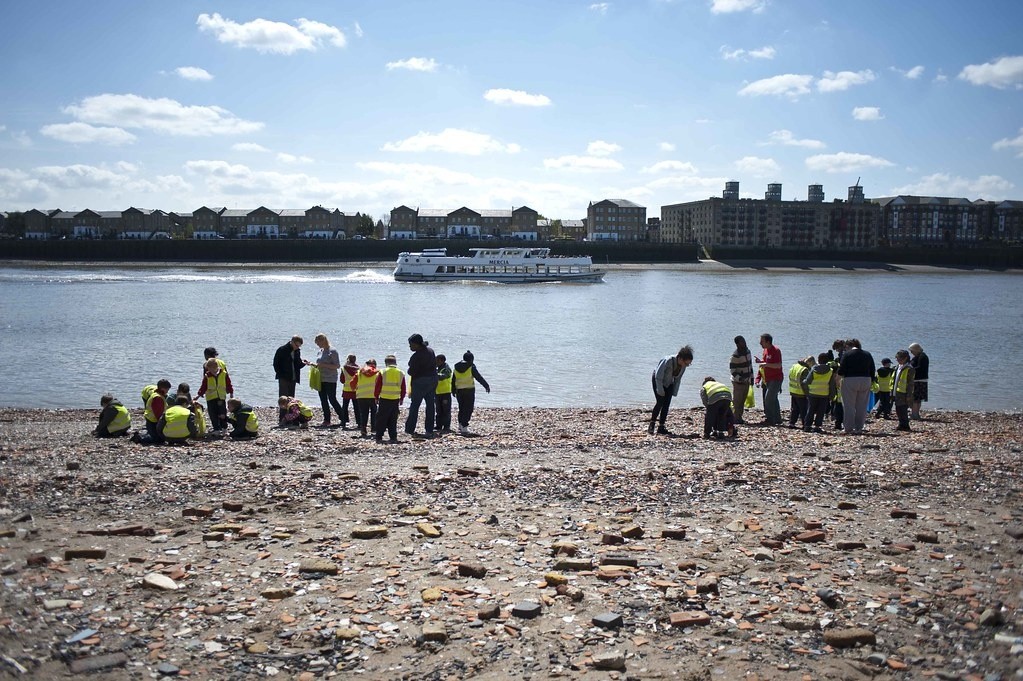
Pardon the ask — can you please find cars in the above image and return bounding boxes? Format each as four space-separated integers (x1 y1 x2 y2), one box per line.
351 235 366 240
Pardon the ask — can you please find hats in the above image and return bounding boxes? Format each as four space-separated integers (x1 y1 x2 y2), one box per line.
463 350 474 362
882 358 893 364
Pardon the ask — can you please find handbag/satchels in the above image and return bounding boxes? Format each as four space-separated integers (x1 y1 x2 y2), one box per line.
745 384 755 408
309 365 322 391
407 375 412 398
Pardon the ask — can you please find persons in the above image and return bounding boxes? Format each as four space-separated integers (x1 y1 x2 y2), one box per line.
700 333 929 440
648 345 694 434
90 334 491 444
549 254 583 258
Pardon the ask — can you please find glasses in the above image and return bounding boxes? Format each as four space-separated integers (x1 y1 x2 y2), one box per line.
684 360 692 367
294 341 300 347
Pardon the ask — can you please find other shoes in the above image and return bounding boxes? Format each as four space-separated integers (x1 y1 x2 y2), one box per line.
657 426 668 434
788 423 796 429
834 421 844 430
704 433 710 439
875 413 879 419
884 414 891 420
458 423 470 434
912 413 923 420
648 422 655 433
891 418 910 431
321 420 330 426
803 426 826 433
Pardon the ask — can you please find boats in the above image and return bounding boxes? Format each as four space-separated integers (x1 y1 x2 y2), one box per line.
393 247 607 281
